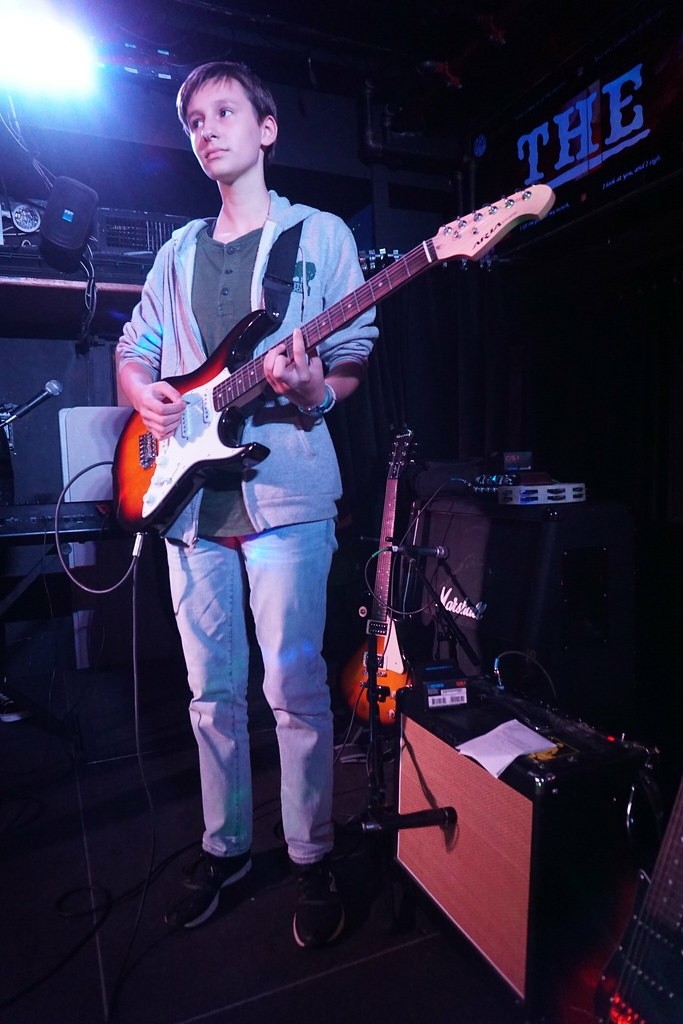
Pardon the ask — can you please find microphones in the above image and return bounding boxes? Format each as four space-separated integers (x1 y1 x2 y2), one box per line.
391 545 449 560
0 379 63 428
358 806 458 834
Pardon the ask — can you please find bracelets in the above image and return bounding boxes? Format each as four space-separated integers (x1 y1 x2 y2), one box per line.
320 383 336 414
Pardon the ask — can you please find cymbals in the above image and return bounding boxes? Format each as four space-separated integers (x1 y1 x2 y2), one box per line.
496 482 588 506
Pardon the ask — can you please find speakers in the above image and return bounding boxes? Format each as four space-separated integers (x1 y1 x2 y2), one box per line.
391 498 660 1008
41 176 99 251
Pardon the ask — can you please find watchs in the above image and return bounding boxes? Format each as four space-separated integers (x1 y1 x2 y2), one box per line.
297 390 329 419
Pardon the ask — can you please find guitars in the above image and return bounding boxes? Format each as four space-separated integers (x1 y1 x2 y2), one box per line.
337 427 419 729
110 181 556 540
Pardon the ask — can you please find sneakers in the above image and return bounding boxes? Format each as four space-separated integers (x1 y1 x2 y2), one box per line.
164 848 252 930
291 854 347 946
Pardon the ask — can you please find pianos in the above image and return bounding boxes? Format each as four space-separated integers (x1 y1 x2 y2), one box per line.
0 499 129 544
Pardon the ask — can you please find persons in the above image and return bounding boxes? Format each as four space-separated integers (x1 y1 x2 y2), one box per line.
116 61 380 947
